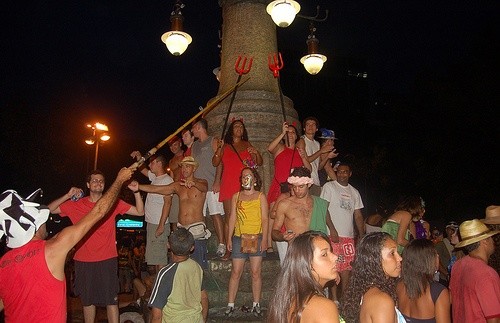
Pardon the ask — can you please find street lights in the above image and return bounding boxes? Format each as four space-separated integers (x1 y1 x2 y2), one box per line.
82 120 113 172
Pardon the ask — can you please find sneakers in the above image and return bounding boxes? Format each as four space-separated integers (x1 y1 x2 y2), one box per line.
215 243 227 257
224 304 236 320
251 302 264 320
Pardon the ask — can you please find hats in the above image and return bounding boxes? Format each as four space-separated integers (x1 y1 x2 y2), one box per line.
318 128 338 139
430 225 443 233
480 205 500 225
177 156 199 167
454 218 500 247
445 222 458 229
0 190 50 249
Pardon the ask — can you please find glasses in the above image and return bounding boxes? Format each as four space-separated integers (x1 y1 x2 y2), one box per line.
431 232 439 236
448 226 455 231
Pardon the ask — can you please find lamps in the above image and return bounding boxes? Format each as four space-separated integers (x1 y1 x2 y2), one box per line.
160 0 193 57
266 0 300 28
299 21 327 77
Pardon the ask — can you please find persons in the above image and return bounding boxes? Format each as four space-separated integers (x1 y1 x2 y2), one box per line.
48 170 145 323
263 230 346 323
0 167 134 323
146 228 208 323
449 219 500 323
393 239 451 323
130 116 500 317
338 232 407 323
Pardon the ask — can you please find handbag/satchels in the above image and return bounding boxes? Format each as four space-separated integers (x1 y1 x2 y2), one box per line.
280 182 290 193
184 221 209 240
239 233 259 254
242 157 258 169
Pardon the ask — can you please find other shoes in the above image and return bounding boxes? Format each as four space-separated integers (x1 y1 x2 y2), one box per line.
120 302 144 316
